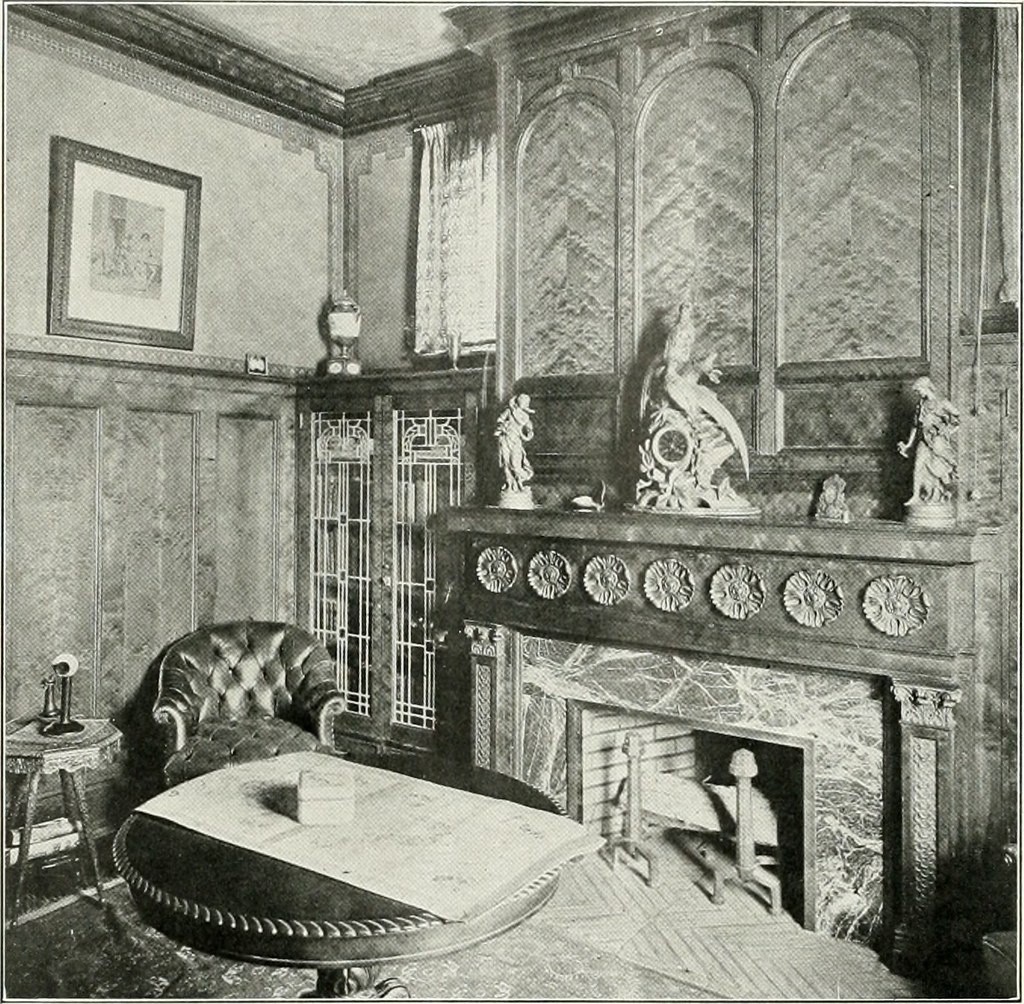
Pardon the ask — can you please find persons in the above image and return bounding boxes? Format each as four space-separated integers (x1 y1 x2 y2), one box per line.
897 377 962 505
495 393 534 491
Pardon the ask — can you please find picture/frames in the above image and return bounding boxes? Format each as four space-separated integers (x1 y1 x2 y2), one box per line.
46 136 203 351
246 352 267 375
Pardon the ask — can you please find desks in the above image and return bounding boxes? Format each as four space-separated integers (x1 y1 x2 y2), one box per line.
112 751 567 998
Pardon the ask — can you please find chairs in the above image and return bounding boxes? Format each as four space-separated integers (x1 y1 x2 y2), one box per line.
151 621 347 789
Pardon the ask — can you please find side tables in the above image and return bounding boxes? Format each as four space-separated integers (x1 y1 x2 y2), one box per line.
4 715 124 930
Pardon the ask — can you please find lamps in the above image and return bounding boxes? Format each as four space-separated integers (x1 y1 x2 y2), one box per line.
319 286 364 376
